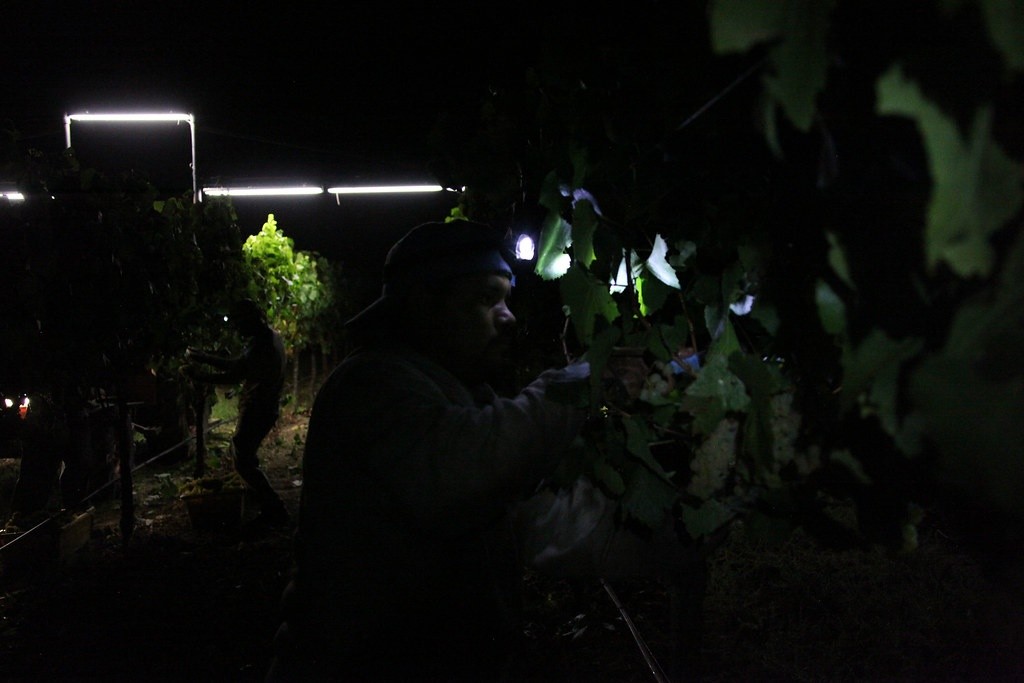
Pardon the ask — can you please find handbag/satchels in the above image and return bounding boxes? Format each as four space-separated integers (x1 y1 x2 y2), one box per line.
516 470 683 580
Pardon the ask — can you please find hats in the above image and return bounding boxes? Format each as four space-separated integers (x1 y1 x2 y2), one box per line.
344 222 513 331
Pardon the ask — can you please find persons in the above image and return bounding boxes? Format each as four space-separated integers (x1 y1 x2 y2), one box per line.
273 219 597 683
185 300 284 516
